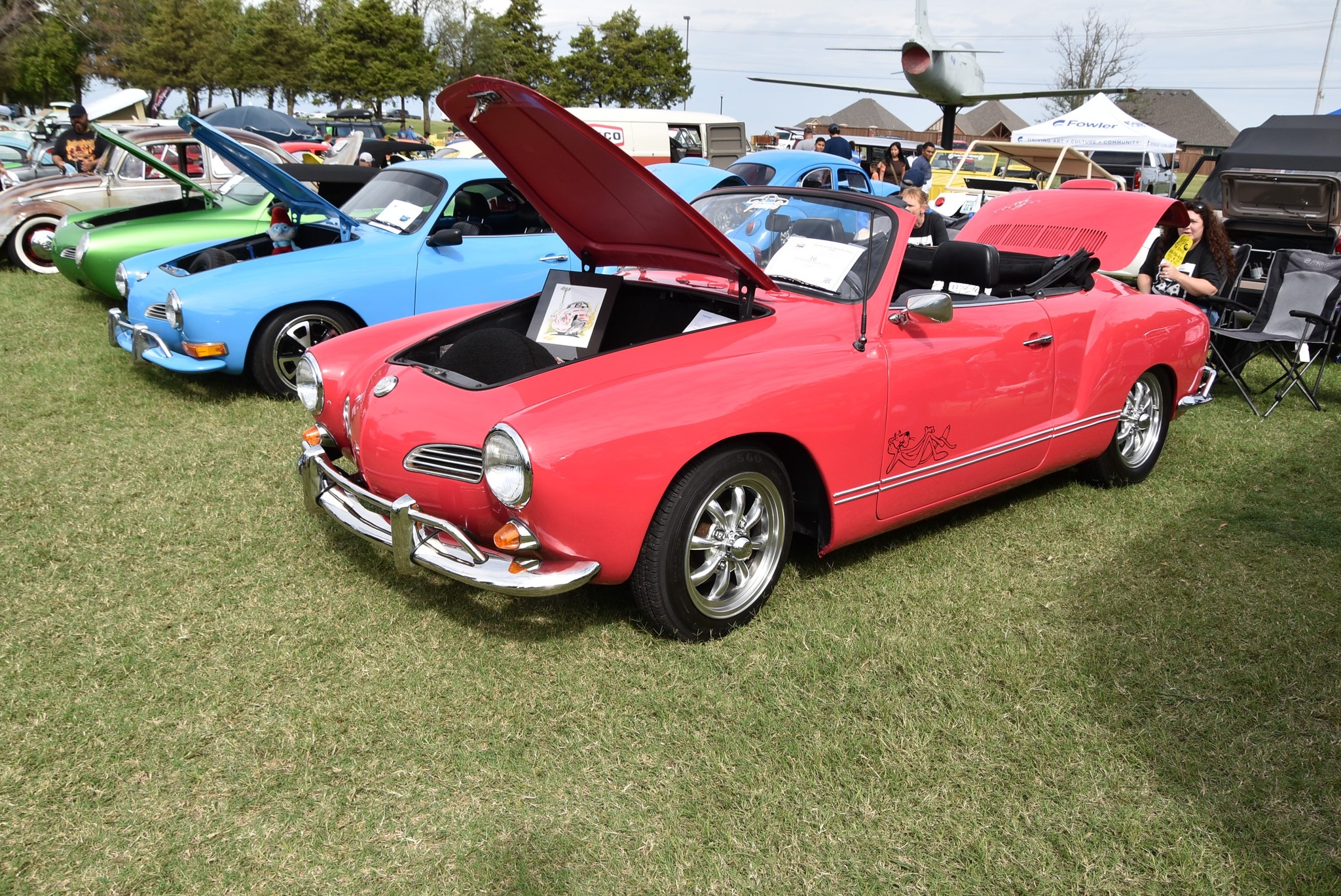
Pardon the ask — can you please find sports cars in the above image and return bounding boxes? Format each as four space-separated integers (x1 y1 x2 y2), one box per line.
52 121 514 312
108 113 760 401
296 73 1216 648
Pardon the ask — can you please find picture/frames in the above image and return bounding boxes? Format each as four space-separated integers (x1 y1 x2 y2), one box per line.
525 269 624 361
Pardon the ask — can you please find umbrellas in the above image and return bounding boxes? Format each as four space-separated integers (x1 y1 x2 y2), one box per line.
203 104 319 145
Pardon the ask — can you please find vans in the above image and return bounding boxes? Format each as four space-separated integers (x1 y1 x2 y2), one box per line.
792 134 953 188
432 108 746 172
766 126 818 150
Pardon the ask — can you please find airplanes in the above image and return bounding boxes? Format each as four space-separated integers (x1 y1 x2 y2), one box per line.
745 1 1145 150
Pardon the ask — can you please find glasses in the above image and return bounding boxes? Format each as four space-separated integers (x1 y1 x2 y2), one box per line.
1183 199 1205 209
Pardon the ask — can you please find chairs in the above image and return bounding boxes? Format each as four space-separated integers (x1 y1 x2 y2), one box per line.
516 203 549 234
895 241 1000 304
790 218 863 300
450 191 492 236
1204 249 1341 419
1118 244 1251 384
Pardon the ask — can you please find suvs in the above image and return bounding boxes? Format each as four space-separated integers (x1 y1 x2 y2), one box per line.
1168 115 1341 357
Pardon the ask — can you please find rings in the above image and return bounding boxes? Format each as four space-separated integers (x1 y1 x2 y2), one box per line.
1165 271 1168 275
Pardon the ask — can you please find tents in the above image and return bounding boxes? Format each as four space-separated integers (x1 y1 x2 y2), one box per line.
1010 92 1177 196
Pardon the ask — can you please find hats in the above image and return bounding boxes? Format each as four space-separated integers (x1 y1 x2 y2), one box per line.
69 105 86 117
828 123 839 131
359 152 375 162
902 169 927 187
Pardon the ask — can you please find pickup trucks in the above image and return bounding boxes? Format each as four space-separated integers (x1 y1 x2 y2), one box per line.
1087 151 1176 198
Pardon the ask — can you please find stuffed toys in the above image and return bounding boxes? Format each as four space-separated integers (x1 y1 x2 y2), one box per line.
266 207 303 255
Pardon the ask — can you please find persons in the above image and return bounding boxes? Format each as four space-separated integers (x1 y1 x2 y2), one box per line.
0 158 8 191
397 125 454 145
359 152 376 167
856 146 867 161
849 140 861 166
823 123 853 186
870 163 879 181
52 104 109 175
321 134 332 157
912 141 936 203
892 168 930 210
886 186 950 248
880 142 906 186
806 137 828 186
794 128 815 151
1138 200 1237 327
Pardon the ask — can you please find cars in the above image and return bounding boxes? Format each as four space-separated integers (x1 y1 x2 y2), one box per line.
0 86 492 176
0 126 320 276
717 150 902 267
952 140 974 166
929 152 1047 201
